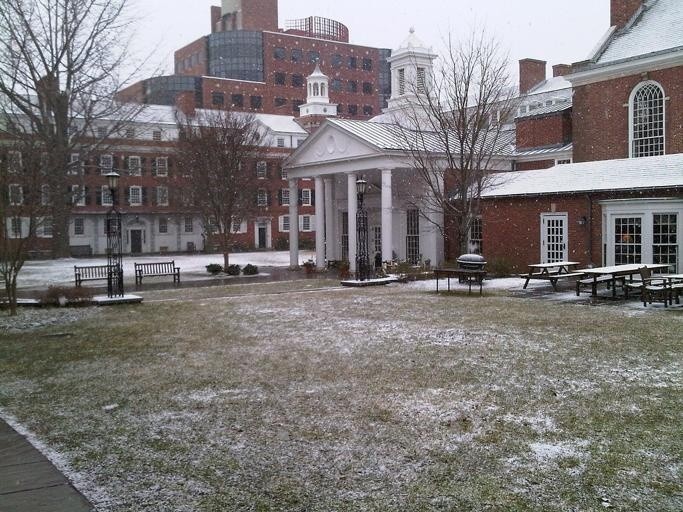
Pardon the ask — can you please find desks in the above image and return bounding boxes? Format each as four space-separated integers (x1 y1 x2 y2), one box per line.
523 261 581 293
571 264 672 300
662 274 683 304
435 268 487 296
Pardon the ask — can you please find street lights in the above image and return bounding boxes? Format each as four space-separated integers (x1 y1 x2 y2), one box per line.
353 176 377 282
100 168 128 300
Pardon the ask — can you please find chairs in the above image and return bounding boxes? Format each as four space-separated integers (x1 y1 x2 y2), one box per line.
671 277 683 304
638 265 672 308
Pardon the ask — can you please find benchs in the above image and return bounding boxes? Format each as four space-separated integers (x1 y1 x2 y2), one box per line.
74 263 120 287
548 272 585 277
134 260 180 285
519 270 584 279
624 280 679 300
575 275 625 296
